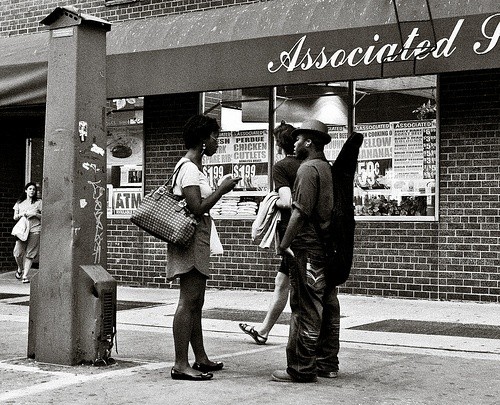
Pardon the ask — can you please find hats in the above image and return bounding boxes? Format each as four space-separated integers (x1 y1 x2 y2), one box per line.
293 118 333 145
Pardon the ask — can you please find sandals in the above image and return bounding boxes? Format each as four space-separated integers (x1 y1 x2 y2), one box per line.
236 320 267 344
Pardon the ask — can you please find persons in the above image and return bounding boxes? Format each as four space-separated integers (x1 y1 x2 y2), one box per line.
239 125 301 345
13 182 43 284
165 114 243 380
271 120 340 383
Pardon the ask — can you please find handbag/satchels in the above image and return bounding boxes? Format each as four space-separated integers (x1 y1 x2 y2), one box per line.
130 159 200 247
11 216 31 241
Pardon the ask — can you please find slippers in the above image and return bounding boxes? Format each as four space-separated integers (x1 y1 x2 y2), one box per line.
13 269 30 284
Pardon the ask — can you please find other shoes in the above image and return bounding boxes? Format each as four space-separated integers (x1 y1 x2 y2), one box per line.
171 361 225 381
271 366 316 384
318 368 338 378
